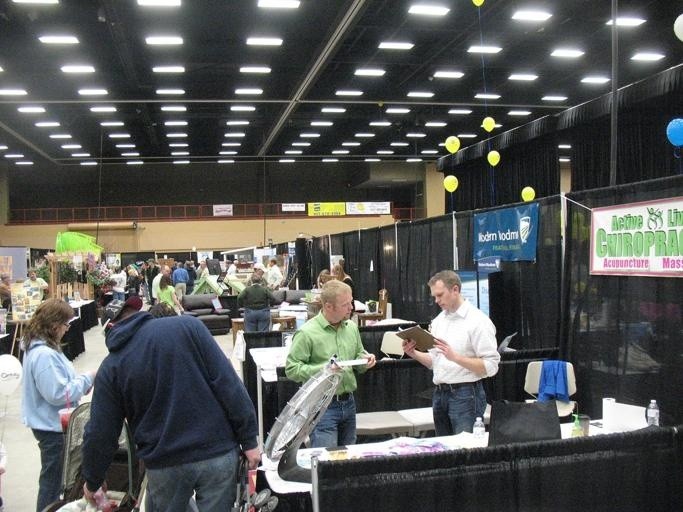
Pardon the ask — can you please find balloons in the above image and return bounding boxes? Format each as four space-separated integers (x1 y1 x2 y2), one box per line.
444 135 460 155
483 117 495 133
673 13 683 42
522 186 535 202
472 0 485 7
666 118 683 148
487 150 501 168
444 174 459 193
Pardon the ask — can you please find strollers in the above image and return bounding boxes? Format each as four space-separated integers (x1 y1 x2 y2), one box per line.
36 400 148 512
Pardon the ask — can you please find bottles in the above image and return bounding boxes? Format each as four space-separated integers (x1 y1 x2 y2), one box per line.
646 399 660 429
472 416 487 441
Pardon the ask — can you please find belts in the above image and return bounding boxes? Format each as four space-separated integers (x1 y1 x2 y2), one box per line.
333 393 349 402
437 382 476 392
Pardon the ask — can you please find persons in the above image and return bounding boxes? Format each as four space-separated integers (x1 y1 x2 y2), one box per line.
402 270 500 437
21 298 112 511
108 260 284 333
286 263 377 449
0 266 49 314
80 294 261 509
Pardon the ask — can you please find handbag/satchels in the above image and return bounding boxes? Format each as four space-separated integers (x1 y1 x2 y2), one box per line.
488 400 561 446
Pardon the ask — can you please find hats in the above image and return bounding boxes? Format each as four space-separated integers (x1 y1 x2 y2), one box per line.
101 296 142 326
253 263 267 273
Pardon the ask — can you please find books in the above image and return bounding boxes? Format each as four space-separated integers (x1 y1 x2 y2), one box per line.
397 326 436 352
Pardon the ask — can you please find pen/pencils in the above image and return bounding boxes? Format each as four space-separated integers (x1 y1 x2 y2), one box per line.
334 361 345 372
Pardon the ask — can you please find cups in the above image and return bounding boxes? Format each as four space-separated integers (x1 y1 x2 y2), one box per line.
58 407 76 436
578 414 590 437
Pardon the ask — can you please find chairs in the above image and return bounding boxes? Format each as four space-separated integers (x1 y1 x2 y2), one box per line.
1 354 23 418
